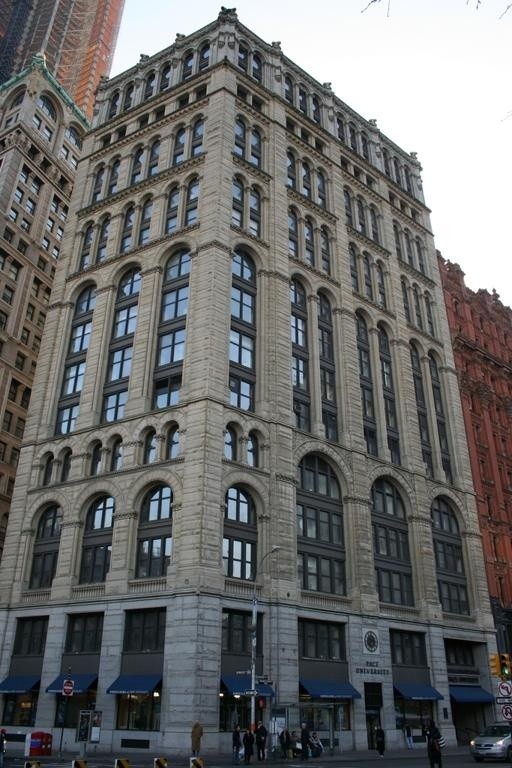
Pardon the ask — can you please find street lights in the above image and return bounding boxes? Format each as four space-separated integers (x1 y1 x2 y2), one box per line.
250 546 284 735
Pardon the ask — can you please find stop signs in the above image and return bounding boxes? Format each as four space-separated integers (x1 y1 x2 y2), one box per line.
62 680 75 696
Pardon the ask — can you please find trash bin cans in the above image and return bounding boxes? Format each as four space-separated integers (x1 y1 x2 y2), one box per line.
30 731 53 756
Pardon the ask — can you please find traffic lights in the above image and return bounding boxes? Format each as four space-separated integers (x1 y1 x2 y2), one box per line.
488 652 512 682
257 696 267 709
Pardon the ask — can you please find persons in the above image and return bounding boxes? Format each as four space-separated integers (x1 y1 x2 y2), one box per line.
425 719 446 768
232 721 268 765
191 720 202 756
376 725 385 757
280 723 325 761
402 720 414 752
0 728 7 753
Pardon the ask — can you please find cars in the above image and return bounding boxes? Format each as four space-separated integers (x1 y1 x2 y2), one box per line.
470 719 512 762
